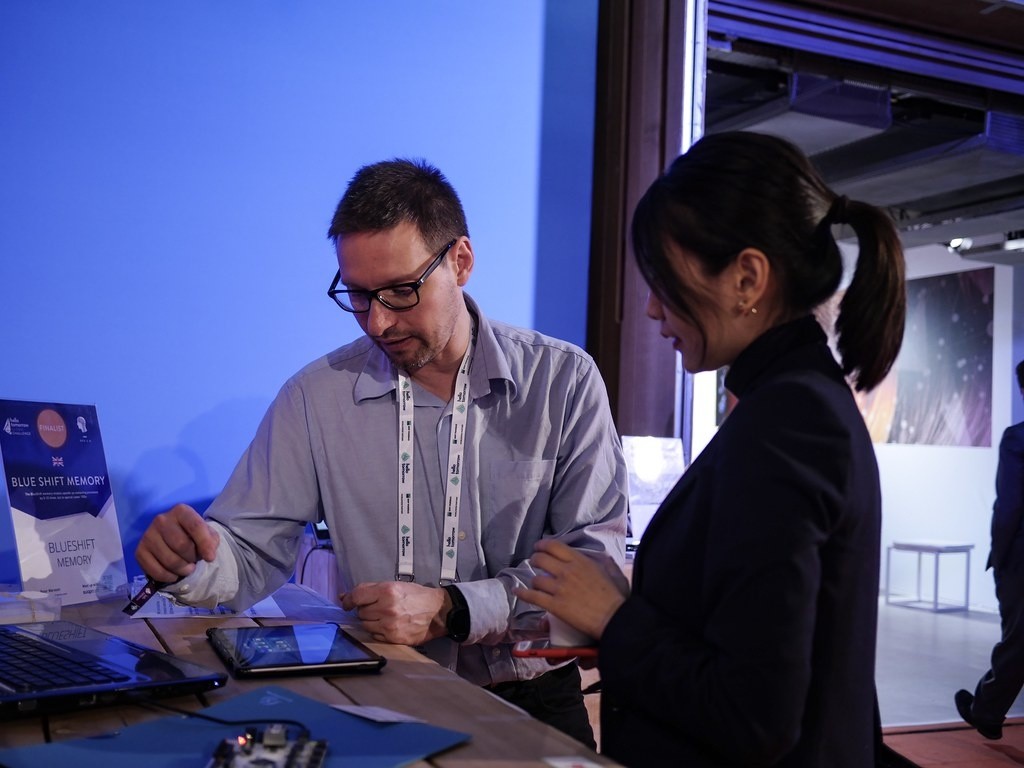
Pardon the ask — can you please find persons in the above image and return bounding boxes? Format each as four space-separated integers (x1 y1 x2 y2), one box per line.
134 156 627 752
954 361 1024 739
512 130 908 768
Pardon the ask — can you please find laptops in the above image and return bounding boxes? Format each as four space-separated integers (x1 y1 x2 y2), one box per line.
0 619 229 722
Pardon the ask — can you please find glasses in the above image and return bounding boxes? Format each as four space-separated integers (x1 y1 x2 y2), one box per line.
328 238 456 314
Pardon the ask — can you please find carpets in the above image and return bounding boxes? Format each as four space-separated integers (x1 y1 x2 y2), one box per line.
881 715 1024 768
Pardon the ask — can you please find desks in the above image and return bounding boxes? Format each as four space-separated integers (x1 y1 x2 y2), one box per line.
0 582 626 768
885 542 974 613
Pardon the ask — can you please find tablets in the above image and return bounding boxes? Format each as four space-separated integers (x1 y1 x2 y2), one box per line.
207 624 388 681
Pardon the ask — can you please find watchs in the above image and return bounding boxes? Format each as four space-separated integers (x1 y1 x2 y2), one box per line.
442 584 470 647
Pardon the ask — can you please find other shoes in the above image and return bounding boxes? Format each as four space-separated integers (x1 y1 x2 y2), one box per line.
955 689 1004 740
